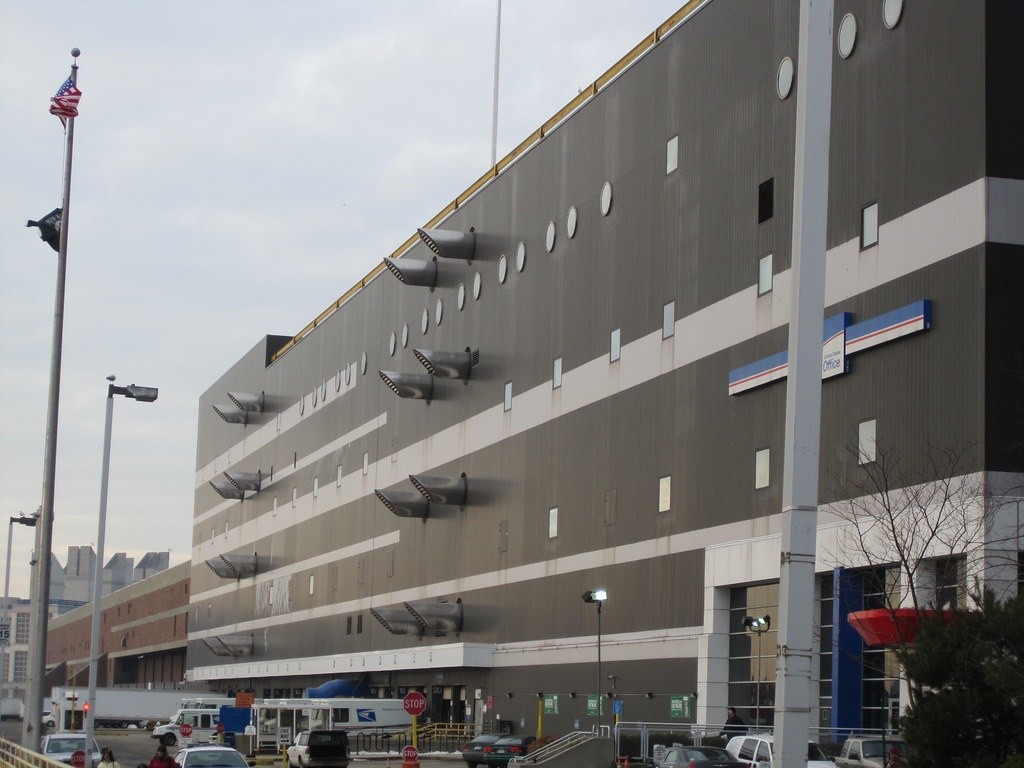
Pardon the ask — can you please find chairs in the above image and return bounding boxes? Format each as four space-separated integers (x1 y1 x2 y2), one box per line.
51 742 61 753
76 741 85 750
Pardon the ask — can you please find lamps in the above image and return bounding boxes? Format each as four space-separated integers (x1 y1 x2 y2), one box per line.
605 692 612 698
569 693 576 698
506 692 512 698
690 693 697 698
536 692 543 698
645 692 653 698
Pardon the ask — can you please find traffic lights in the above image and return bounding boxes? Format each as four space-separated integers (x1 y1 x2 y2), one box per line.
83 704 89 712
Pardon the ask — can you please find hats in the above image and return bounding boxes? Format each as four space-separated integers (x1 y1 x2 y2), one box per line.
728 707 736 713
157 744 167 752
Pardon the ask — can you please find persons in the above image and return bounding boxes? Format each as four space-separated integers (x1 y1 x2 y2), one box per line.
136 763 148 768
885 746 925 768
97 746 120 768
149 745 180 768
718 707 749 739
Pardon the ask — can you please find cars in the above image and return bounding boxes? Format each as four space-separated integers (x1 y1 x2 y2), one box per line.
462 732 503 768
483 734 537 768
41 733 102 768
41 711 58 728
173 745 249 768
835 734 910 768
645 747 756 768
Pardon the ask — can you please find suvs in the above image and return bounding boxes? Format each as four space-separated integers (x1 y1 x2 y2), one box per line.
286 729 351 768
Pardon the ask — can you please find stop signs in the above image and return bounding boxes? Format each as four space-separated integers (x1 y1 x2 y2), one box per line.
180 724 193 737
72 751 85 768
404 692 426 717
402 746 418 762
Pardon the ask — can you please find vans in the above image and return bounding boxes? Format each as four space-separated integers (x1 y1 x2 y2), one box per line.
150 707 222 746
725 733 837 768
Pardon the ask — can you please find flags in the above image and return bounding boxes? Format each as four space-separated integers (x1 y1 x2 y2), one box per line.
27 200 65 254
49 75 82 129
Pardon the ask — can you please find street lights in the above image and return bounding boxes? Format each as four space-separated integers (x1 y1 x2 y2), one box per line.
81 376 159 745
1 509 38 650
582 590 607 738
742 615 770 734
